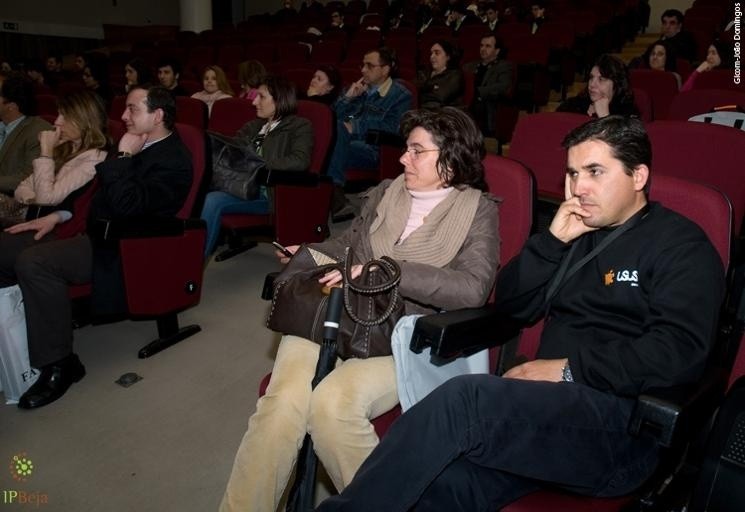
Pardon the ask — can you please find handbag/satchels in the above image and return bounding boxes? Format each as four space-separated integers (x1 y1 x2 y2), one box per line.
207 127 272 203
0 191 42 230
264 239 406 362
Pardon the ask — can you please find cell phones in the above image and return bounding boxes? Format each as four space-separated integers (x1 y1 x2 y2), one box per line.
271 239 295 260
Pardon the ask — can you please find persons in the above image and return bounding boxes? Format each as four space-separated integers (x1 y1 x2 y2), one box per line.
1 0 744 512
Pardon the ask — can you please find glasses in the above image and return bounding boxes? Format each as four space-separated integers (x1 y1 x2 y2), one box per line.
359 62 385 70
405 146 442 156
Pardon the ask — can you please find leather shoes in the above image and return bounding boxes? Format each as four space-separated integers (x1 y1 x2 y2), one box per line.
16 353 87 411
